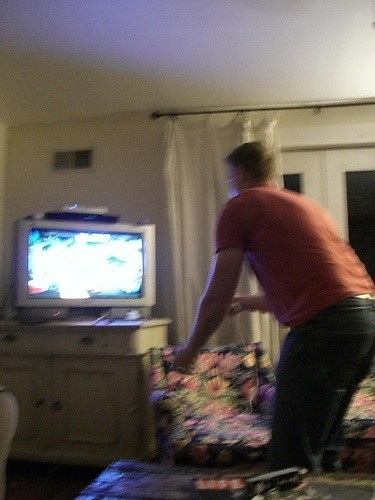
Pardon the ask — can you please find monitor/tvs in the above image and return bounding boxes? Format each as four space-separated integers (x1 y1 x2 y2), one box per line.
16 219 156 306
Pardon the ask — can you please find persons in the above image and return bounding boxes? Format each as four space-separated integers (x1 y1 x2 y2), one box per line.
172 140 375 469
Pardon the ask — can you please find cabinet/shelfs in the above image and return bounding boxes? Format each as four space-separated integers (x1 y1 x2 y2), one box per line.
1 318 174 469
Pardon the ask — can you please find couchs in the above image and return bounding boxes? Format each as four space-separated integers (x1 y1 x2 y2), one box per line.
148 342 278 464
